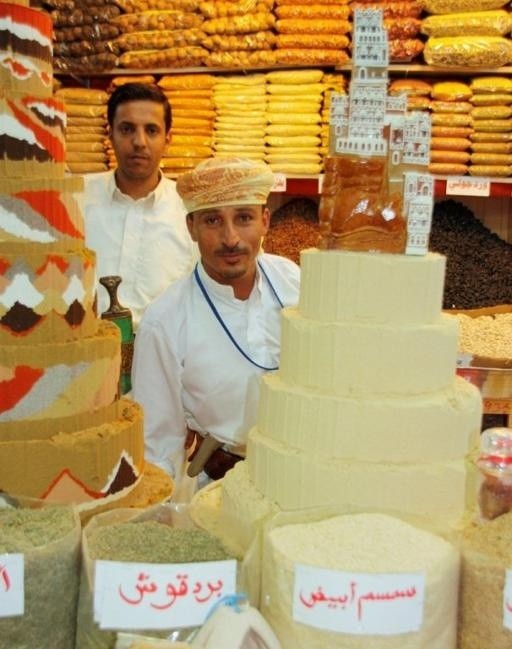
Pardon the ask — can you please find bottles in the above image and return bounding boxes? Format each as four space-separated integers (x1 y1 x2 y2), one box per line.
489 437 512 475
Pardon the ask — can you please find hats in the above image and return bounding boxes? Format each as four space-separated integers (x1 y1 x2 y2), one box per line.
176 156 273 213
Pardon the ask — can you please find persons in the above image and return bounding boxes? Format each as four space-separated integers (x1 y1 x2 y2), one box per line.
130 152 302 483
69 81 201 338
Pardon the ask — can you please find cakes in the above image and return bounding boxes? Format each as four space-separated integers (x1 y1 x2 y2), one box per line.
244 246 483 521
0 1 176 527
